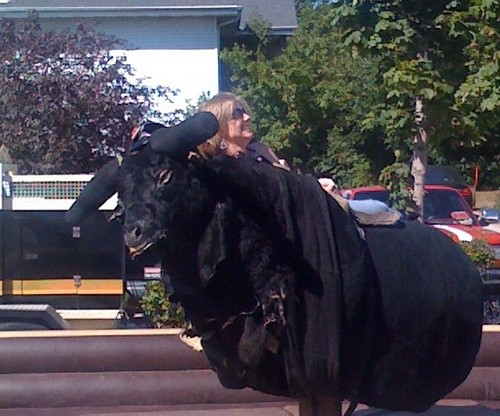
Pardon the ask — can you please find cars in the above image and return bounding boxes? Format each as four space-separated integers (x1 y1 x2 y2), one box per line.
345 185 500 304
423 165 474 212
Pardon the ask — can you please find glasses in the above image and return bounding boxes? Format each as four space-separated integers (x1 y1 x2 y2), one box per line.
230 108 246 121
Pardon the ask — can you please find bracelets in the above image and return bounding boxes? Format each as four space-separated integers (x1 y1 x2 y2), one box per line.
218 136 227 153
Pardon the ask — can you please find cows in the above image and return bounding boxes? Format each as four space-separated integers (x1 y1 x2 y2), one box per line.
67 112 484 416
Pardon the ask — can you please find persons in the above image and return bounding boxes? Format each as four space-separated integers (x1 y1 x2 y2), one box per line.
200 91 418 222
